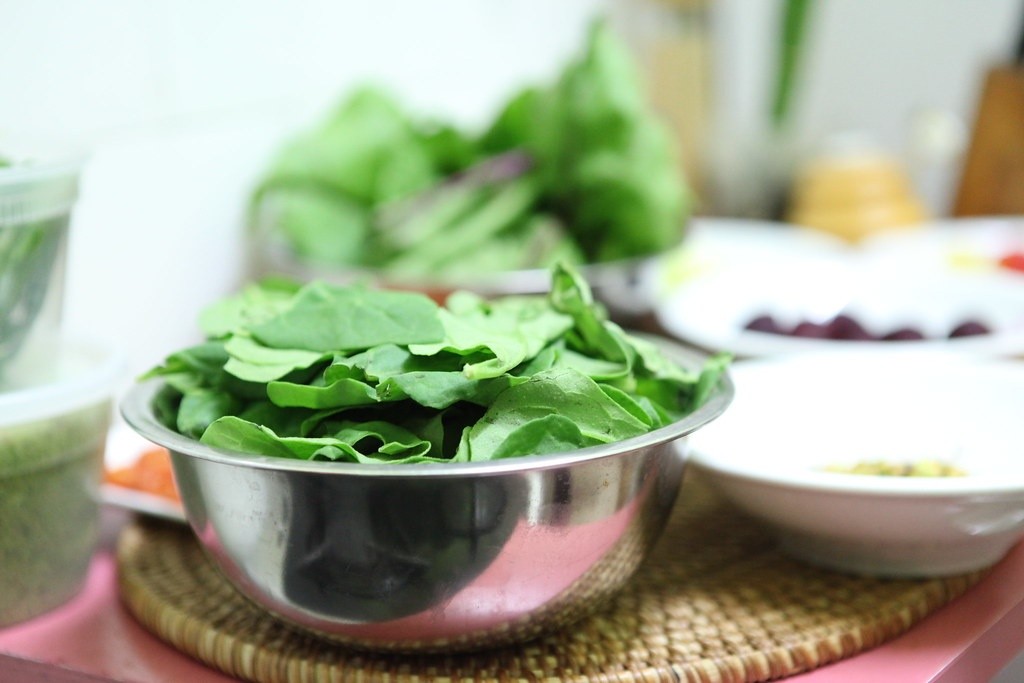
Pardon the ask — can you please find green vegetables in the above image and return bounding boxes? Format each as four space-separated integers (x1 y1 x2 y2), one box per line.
123 19 735 474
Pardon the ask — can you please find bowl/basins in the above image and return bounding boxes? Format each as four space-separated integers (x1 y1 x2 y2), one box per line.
118 213 1024 655
